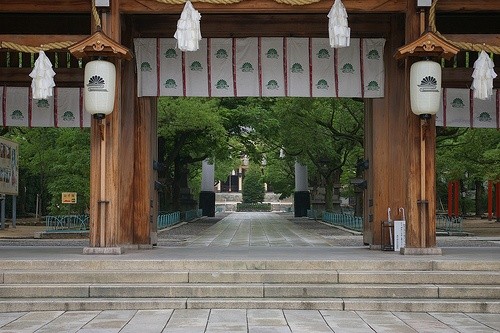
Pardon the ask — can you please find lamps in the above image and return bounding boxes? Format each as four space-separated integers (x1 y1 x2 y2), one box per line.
84 61 117 124
408 61 442 120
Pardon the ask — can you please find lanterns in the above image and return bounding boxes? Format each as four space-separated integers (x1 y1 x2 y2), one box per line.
83 55 115 126
409 58 442 122
